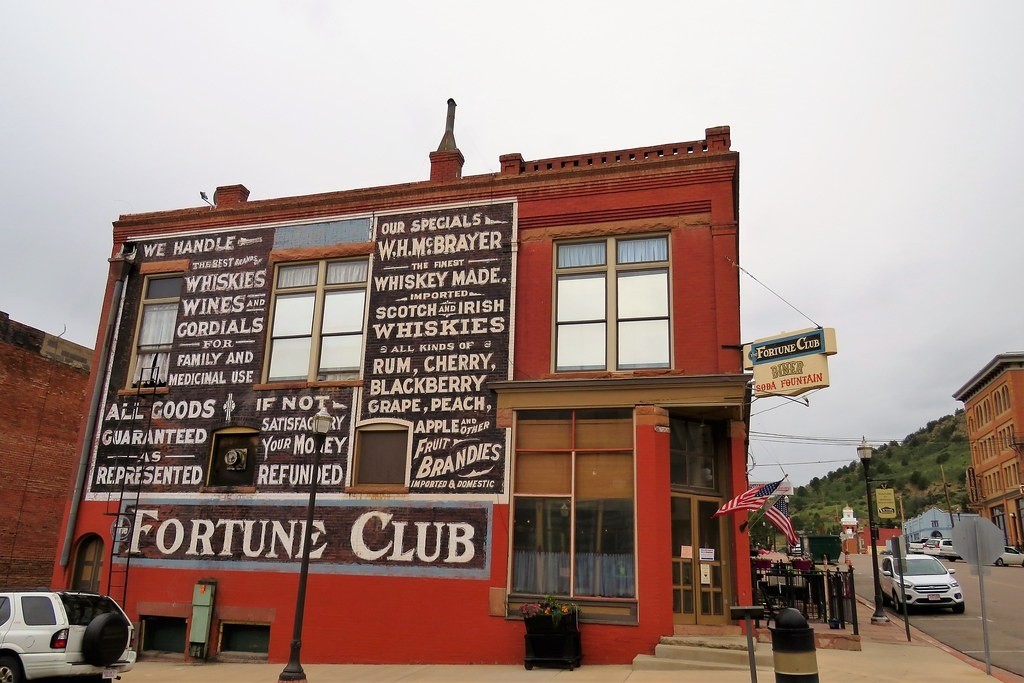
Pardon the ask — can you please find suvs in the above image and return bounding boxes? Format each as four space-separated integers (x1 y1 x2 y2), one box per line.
923 538 963 562
878 554 966 614
0 592 137 683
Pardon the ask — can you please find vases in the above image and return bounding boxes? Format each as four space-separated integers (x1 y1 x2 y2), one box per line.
524 611 584 669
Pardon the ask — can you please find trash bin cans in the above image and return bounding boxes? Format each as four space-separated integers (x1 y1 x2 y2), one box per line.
768 609 819 683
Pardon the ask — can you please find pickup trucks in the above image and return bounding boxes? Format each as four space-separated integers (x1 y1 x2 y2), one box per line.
909 538 930 553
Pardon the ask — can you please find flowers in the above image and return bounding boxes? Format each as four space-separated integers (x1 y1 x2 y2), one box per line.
520 595 583 619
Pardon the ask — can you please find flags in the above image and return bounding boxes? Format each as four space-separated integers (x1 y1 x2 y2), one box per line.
706 481 779 519
762 495 800 548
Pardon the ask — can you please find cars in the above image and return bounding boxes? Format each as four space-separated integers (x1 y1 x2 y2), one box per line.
996 546 1024 567
787 538 801 555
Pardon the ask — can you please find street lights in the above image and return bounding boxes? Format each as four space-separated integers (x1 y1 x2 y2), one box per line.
857 437 892 625
279 407 333 680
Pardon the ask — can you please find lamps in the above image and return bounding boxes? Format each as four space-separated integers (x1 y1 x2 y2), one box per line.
201 191 215 208
